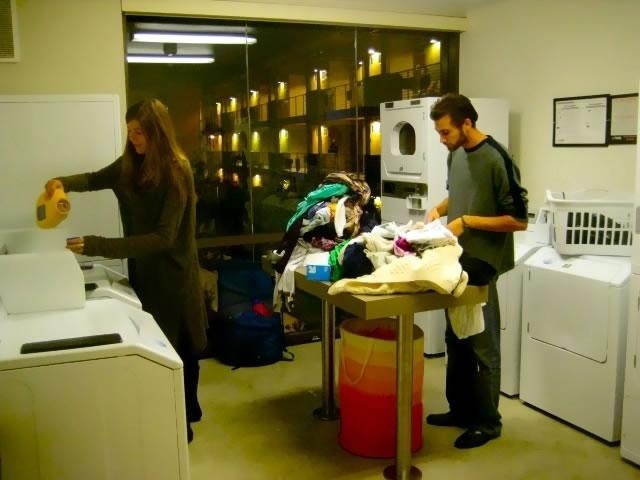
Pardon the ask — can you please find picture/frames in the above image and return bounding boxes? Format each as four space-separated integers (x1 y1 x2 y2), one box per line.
609 93 639 144
553 94 610 147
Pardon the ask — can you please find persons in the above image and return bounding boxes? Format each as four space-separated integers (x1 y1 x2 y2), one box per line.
424 91 530 450
44 97 208 444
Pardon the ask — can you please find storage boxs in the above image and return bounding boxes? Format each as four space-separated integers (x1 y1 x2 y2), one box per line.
338 318 424 458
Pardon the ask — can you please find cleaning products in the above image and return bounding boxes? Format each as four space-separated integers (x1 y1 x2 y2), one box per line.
35 183 73 229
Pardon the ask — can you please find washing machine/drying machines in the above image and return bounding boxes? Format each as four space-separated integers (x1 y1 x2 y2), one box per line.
497 223 551 396
1 298 189 480
519 246 632 442
620 273 640 465
380 97 508 358
1 230 141 315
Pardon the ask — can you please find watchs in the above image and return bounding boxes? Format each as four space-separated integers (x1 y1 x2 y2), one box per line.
460 215 469 233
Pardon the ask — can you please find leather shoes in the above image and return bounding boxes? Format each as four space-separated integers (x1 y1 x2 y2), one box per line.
455 427 495 448
427 411 468 426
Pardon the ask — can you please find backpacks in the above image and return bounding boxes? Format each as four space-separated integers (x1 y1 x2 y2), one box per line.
225 306 295 371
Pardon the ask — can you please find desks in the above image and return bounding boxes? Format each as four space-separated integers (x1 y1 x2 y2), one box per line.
261 254 488 480
